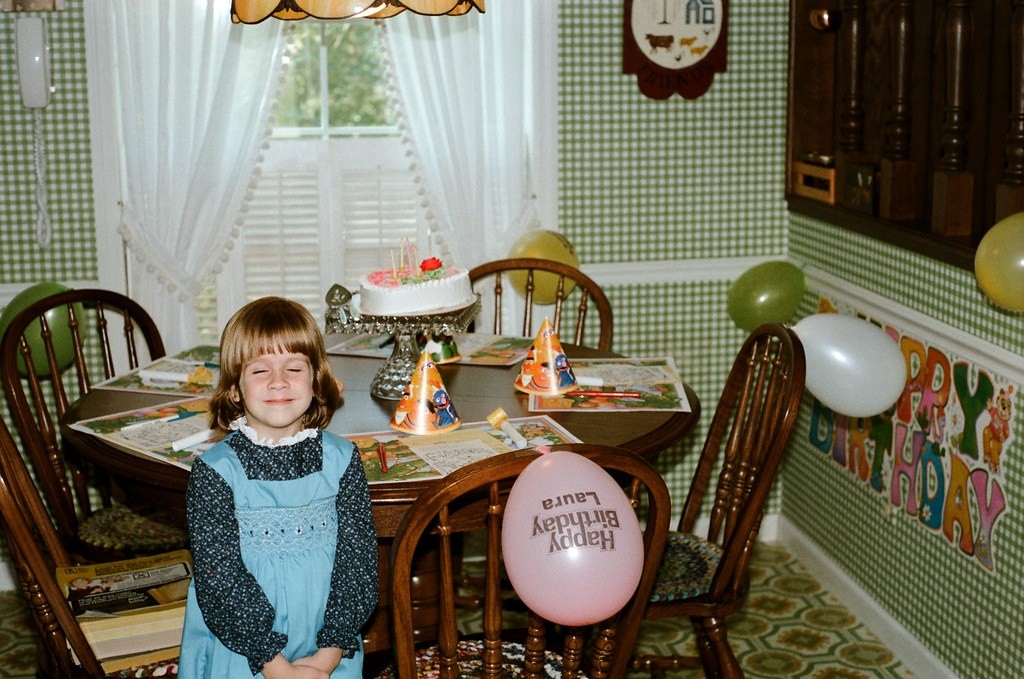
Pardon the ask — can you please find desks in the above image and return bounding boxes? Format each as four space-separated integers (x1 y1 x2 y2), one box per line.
65 335 701 648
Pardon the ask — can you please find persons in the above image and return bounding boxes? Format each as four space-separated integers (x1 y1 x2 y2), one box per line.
66 574 112 615
175 294 379 679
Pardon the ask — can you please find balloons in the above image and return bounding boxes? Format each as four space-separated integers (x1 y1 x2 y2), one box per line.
729 262 803 331
506 228 579 306
501 449 644 626
790 313 907 418
974 212 1024 316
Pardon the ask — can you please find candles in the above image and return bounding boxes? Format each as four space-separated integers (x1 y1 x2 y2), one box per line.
413 245 420 275
400 237 403 268
427 233 432 258
405 237 412 270
390 248 397 276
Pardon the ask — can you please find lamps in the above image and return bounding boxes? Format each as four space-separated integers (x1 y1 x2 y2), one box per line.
229 0 486 26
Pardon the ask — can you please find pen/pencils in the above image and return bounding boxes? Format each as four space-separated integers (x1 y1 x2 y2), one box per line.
163 356 220 367
120 411 199 432
566 391 641 398
378 443 388 472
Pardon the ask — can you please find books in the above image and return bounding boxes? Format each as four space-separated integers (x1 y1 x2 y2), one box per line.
56 548 197 666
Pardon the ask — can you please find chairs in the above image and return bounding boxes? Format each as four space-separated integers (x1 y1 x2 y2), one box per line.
465 259 615 350
0 416 182 679
0 290 191 561
369 442 671 679
627 319 807 679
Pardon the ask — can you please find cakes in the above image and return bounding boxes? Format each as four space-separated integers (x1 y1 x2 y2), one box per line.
359 256 473 315
